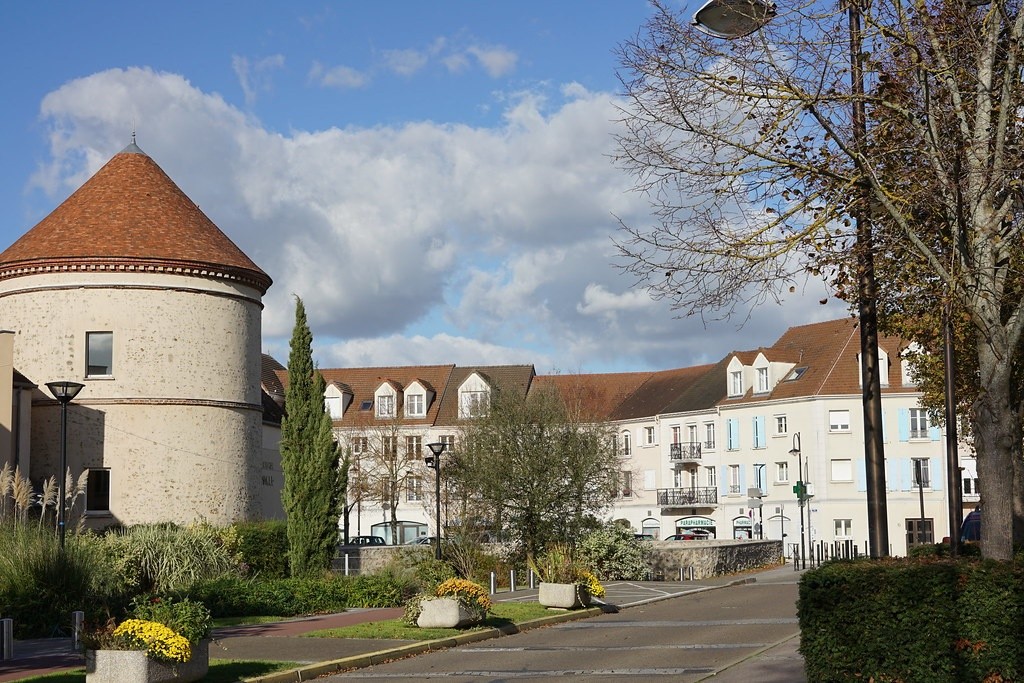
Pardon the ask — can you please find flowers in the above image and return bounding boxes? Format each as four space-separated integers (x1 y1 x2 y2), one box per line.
404 576 493 613
73 596 214 664
527 549 605 601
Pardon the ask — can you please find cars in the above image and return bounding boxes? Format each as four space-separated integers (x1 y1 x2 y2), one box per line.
622 533 655 542
404 536 445 544
350 535 386 545
961 510 981 555
664 532 709 541
468 529 528 553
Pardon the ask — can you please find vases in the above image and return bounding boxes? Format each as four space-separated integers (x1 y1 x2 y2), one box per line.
85 641 209 683
538 581 591 609
413 599 486 629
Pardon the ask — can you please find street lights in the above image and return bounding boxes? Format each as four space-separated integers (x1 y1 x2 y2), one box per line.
789 433 806 569
349 455 361 543
423 441 447 561
45 381 88 565
688 0 888 563
803 456 812 569
